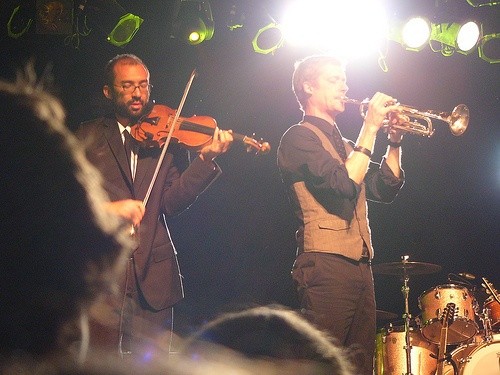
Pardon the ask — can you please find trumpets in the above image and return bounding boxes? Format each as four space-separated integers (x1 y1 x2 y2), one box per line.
342 95 470 138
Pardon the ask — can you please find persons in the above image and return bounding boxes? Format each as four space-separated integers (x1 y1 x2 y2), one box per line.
276 56 407 375
75 53 234 375
173 306 348 375
1 84 139 375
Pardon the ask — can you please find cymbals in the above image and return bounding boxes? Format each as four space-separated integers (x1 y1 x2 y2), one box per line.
376 309 399 321
371 261 443 276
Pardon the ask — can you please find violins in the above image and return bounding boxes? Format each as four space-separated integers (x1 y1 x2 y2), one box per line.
131 102 270 156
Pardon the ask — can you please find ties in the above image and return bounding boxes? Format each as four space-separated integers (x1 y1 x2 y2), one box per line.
332 126 348 162
122 130 134 178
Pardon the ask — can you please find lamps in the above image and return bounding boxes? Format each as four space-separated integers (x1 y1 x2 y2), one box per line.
0 0 500 65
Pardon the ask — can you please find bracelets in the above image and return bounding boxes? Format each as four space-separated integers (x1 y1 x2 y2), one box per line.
354 146 372 157
387 139 403 148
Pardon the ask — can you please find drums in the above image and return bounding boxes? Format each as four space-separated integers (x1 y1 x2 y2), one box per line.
374 325 438 375
478 293 500 330
418 283 478 345
435 329 500 375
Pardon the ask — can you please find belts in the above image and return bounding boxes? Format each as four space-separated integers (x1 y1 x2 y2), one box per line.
360 246 370 258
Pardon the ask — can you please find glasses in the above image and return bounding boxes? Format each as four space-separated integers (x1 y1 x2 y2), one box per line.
112 83 154 97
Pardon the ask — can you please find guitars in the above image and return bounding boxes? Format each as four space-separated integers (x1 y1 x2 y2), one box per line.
436 303 458 375
